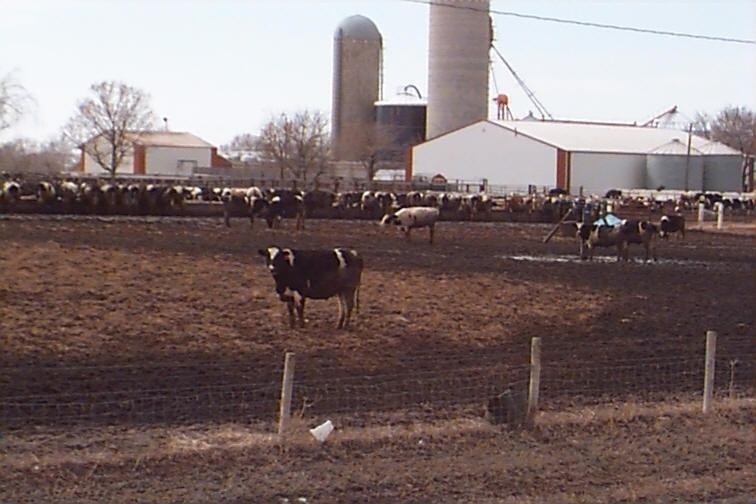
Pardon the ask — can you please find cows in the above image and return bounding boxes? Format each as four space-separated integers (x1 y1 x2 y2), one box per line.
573 214 686 259
215 182 755 229
2 174 207 214
256 242 365 331
379 205 440 244
